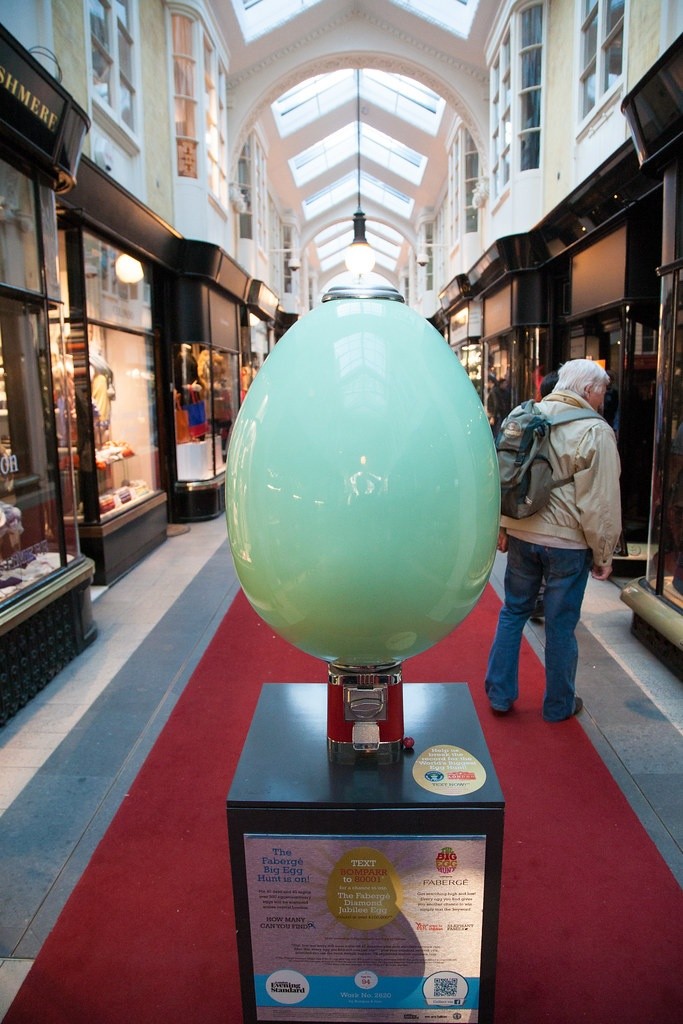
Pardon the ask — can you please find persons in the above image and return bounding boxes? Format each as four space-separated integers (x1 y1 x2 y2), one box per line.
197 349 232 456
89 342 115 430
485 375 504 434
484 358 623 722
175 344 207 441
498 378 511 411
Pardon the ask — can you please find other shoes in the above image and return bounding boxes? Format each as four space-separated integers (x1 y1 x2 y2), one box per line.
529 615 545 625
494 704 515 718
574 696 583 714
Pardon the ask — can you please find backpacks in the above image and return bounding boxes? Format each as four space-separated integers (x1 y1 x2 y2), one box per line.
495 397 606 521
